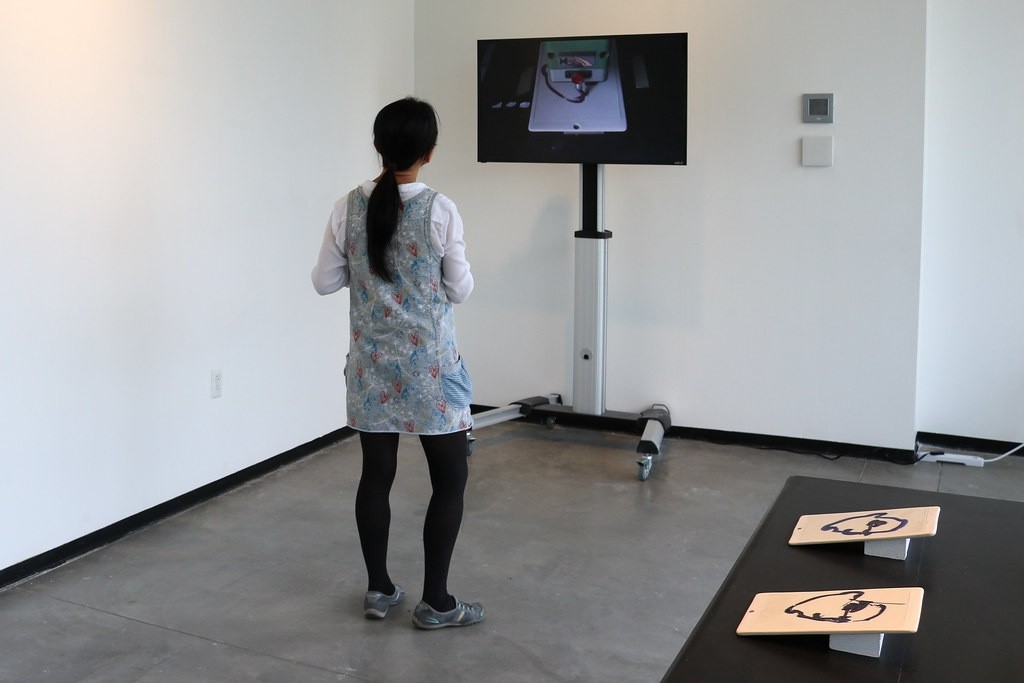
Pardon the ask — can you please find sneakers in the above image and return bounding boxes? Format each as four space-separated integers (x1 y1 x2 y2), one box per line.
412 595 486 630
363 584 403 618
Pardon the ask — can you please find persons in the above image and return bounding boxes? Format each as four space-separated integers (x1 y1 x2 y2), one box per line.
312 96 486 631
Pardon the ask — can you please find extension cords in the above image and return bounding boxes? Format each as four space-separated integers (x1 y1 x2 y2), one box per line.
917 451 984 466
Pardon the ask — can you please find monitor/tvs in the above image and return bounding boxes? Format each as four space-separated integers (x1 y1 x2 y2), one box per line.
475 32 688 166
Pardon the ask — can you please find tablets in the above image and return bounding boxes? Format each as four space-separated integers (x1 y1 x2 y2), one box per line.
789 506 941 545
736 586 924 635
